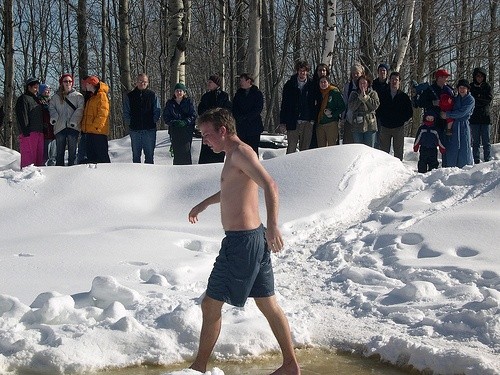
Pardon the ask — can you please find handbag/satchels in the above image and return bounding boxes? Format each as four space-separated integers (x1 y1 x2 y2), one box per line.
363 115 378 133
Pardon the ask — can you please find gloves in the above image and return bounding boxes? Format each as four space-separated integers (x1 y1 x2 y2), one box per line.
413 145 419 152
175 120 187 127
440 148 446 154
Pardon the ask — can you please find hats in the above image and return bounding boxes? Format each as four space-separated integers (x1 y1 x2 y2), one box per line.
39 83 48 94
87 76 99 86
210 75 221 86
377 64 388 73
457 80 471 89
26 78 39 86
442 86 454 96
175 83 186 92
436 69 451 77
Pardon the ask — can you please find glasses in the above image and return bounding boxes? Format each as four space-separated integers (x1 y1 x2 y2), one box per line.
137 80 149 84
477 75 483 78
62 80 73 84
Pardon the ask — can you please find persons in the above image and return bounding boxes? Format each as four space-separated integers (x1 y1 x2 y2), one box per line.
280 60 415 161
15 74 112 168
188 107 301 375
411 66 493 173
163 72 266 165
121 74 162 164
